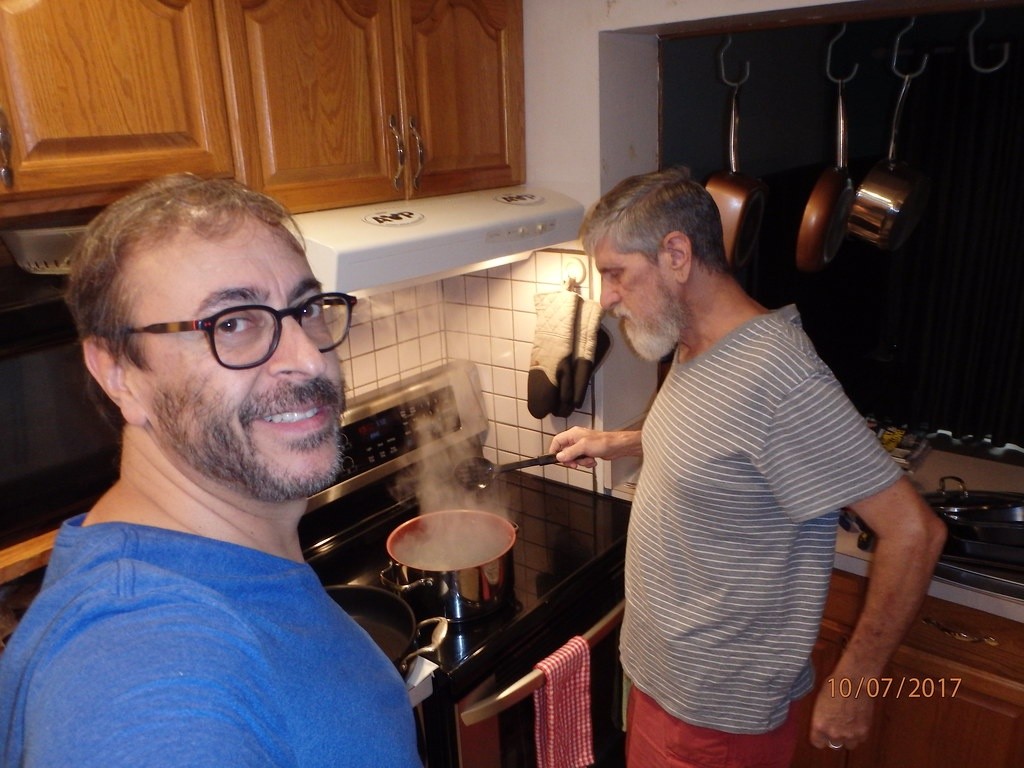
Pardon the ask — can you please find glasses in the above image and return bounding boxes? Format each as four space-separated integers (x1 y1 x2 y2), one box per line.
103 291 358 369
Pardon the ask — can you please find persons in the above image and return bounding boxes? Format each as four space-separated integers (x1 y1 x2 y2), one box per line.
0 173 417 768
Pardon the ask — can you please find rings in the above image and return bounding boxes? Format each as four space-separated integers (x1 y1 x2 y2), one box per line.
828 742 843 749
550 168 947 768
